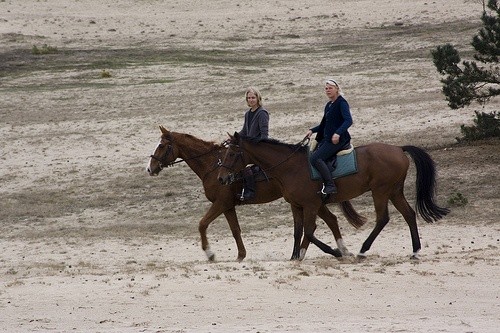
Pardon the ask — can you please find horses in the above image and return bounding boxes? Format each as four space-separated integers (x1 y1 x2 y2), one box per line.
146 126 450 262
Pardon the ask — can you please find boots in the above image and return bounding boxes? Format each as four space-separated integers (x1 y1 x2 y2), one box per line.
313 158 337 194
237 177 257 201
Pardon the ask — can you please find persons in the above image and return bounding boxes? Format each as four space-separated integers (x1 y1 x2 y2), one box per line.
306 79 353 195
213 86 269 205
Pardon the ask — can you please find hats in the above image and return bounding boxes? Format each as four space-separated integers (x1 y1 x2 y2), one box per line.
326 79 339 86
